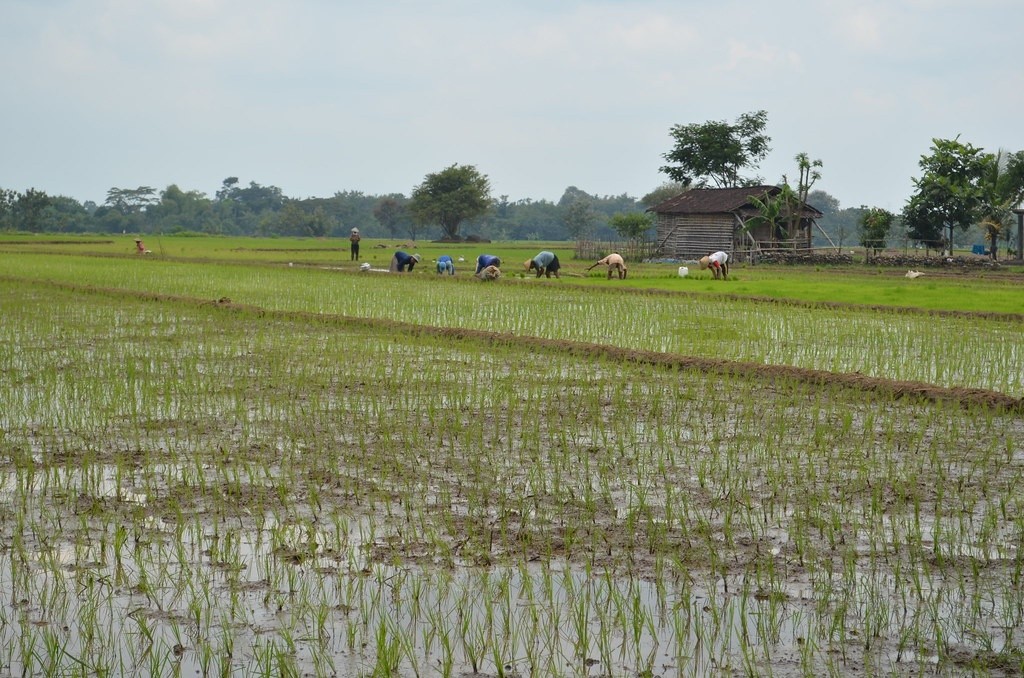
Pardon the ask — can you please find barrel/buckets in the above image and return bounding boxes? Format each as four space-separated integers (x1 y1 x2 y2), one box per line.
678 266 688 277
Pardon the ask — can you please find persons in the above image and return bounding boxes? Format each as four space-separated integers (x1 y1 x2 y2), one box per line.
523 251 561 279
699 251 729 281
474 255 500 280
134 238 144 255
389 251 420 272
584 251 627 280
349 227 360 261
436 255 456 275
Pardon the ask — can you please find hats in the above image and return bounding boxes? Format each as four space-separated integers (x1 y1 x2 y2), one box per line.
494 257 500 267
524 259 531 273
134 238 141 241
412 254 420 263
700 256 709 270
351 227 359 233
439 262 446 272
